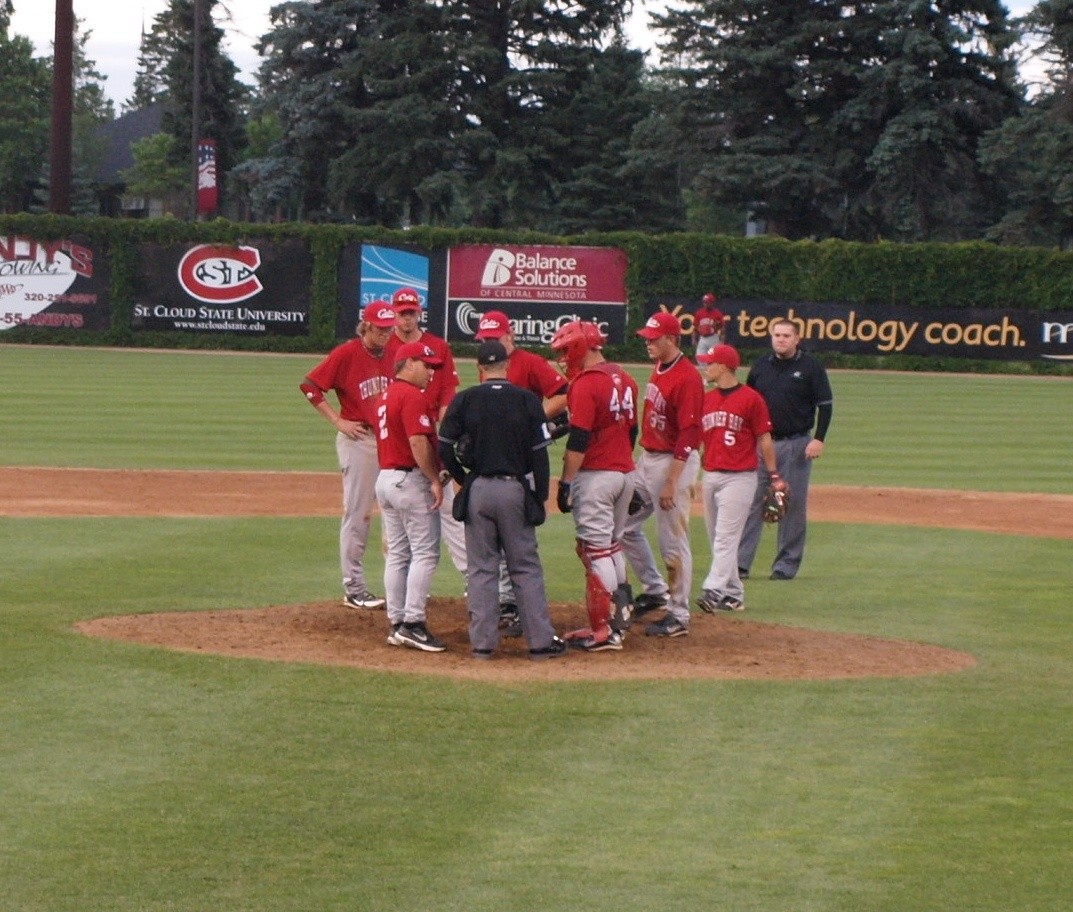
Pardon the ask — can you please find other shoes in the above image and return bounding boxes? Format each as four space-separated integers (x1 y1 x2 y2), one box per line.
472 647 494 657
739 570 749 578
569 630 624 651
498 604 521 636
529 640 568 659
343 592 385 609
769 570 794 582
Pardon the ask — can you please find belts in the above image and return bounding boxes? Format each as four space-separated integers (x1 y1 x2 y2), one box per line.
393 467 412 472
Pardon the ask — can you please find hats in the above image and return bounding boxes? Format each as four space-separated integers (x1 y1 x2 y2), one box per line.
392 288 421 313
696 344 740 368
396 340 445 368
477 342 507 364
364 300 396 328
702 293 715 304
474 311 510 340
636 312 681 340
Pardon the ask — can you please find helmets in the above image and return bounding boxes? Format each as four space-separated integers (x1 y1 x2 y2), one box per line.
550 321 602 353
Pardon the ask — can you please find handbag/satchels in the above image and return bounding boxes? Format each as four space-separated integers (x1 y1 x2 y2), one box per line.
452 474 471 522
521 479 545 527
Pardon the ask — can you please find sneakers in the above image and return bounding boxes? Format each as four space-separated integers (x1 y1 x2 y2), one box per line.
635 590 672 612
387 622 450 653
697 589 744 613
646 616 688 638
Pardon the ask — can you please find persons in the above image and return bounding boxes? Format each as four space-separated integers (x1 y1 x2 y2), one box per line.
299 288 570 637
617 312 704 640
690 292 727 386
695 344 791 617
437 338 570 660
371 341 451 654
549 320 639 652
735 320 833 580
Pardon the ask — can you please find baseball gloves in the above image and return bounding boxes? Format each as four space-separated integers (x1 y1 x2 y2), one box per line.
761 473 793 521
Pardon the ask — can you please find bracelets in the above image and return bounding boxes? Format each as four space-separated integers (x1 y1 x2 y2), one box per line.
330 416 341 424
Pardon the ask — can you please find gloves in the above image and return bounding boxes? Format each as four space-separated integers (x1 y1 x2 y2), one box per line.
557 481 572 513
628 490 644 515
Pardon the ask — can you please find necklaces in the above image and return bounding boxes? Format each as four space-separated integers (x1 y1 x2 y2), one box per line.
360 335 385 358
655 351 684 375
